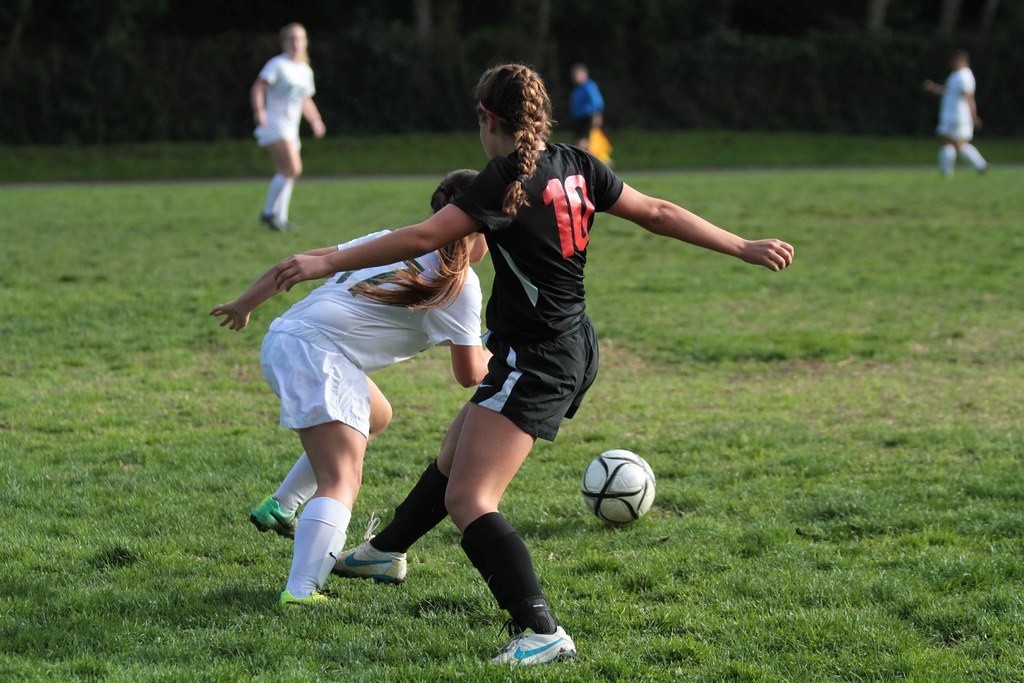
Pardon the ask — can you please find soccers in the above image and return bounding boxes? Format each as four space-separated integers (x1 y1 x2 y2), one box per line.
582 448 658 526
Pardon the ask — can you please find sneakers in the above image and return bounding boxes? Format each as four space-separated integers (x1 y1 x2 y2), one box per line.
278 589 358 609
250 494 299 539
328 512 407 584
494 617 576 666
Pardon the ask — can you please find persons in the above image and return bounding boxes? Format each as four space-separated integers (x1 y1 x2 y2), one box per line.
924 48 989 179
250 22 326 232
570 63 605 150
273 65 795 666
209 169 495 610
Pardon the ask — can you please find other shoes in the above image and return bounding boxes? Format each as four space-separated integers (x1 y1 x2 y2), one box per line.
259 209 285 231
